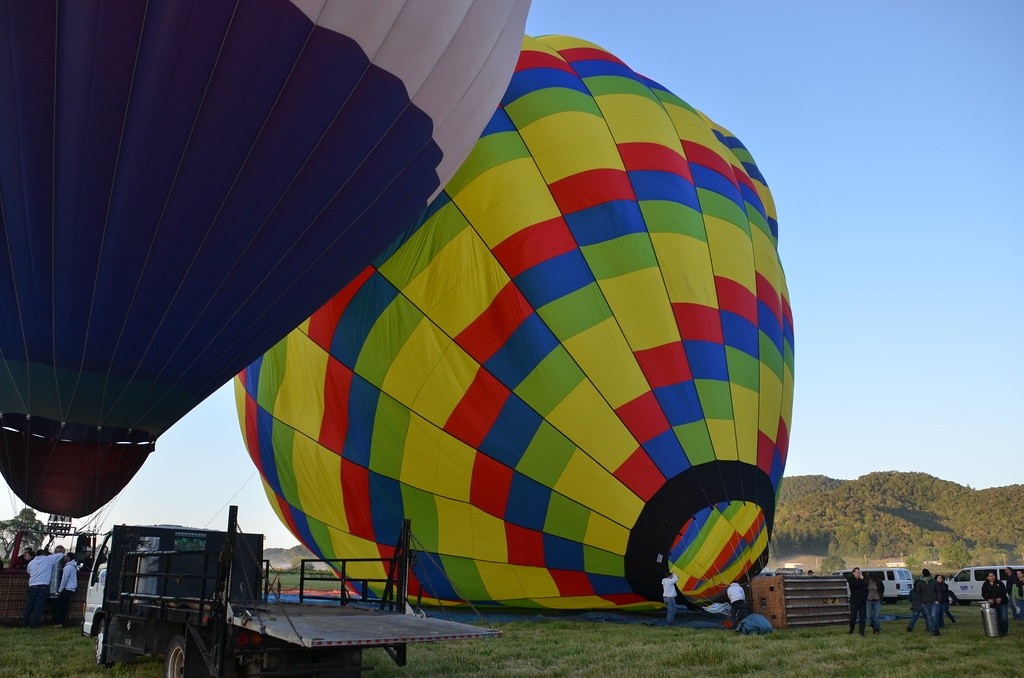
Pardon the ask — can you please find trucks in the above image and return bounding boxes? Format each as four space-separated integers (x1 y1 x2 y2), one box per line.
81 521 505 678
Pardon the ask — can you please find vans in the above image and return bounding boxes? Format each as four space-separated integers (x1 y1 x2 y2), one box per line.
829 566 915 604
945 564 1024 607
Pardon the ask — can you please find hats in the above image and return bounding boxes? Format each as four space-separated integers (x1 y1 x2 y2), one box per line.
922 569 931 577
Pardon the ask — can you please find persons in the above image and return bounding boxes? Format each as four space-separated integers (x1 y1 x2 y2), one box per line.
808 570 813 576
847 567 869 636
906 569 959 636
981 571 1009 636
16 545 65 628
727 580 748 630
1005 567 1021 620
866 574 884 634
662 573 679 624
1011 570 1024 621
54 552 79 629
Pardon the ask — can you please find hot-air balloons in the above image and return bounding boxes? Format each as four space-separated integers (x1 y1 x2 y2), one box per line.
234 30 788 630
0 0 533 626
